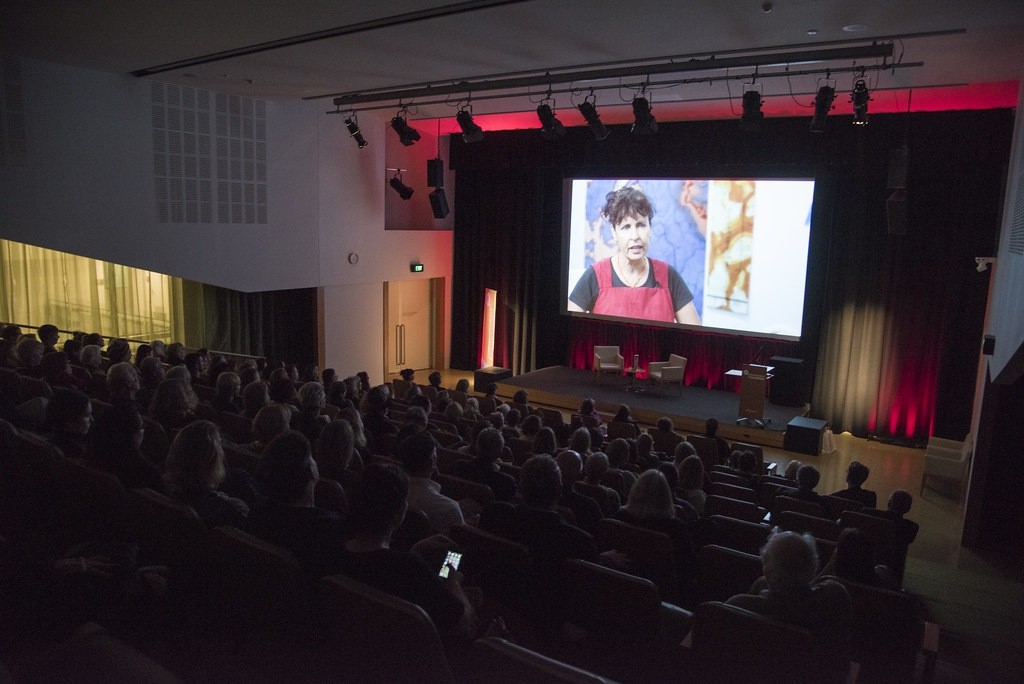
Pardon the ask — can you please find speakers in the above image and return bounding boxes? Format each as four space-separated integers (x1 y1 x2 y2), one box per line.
886 148 911 234
427 159 450 218
983 335 995 355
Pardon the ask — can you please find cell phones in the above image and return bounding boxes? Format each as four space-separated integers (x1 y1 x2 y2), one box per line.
437 550 464 581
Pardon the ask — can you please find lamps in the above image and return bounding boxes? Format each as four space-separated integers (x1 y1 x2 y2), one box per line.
976 256 995 272
736 74 766 131
577 87 613 140
809 70 838 133
392 106 421 147
344 112 368 149
388 167 415 201
630 82 660 136
455 103 486 145
847 69 874 127
536 93 567 142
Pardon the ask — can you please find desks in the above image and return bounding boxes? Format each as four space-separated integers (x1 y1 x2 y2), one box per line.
724 369 774 396
624 367 646 394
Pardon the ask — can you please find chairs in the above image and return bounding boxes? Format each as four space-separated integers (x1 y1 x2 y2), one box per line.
0 346 974 683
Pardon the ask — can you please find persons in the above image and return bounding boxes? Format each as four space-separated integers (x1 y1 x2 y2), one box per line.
0 323 914 684
568 186 699 325
